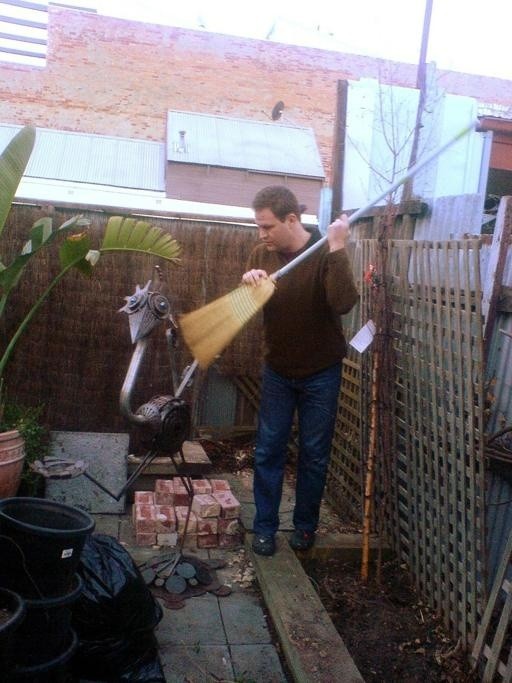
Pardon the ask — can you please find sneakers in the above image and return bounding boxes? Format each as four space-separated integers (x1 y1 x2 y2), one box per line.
251 532 276 557
290 527 316 552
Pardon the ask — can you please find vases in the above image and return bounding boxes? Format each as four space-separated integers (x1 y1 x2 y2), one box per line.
1 493 95 681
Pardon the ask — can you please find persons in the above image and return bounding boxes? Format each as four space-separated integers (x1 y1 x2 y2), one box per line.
236 184 359 557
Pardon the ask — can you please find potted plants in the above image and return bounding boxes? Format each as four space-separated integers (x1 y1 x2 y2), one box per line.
0 124 183 496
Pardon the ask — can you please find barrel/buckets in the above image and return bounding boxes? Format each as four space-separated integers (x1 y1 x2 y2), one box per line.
16 573 83 666
0 497 97 602
9 625 77 681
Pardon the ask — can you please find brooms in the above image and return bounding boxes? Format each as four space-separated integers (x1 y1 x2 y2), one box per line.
177 118 484 369
